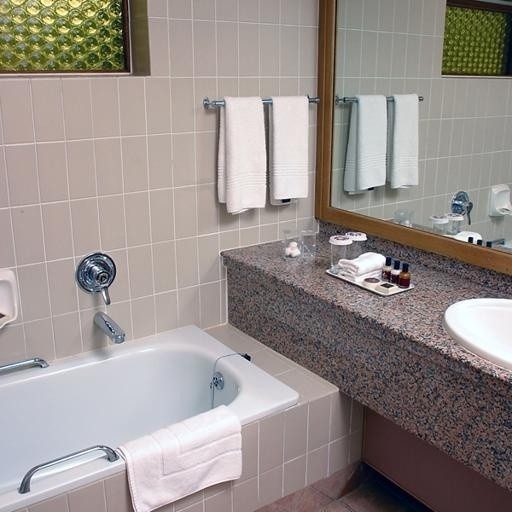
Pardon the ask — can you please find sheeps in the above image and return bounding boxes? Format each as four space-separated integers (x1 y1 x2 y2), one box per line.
94 312 125 344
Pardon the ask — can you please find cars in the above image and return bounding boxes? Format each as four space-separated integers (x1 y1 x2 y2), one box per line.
392 209 413 230
281 229 317 261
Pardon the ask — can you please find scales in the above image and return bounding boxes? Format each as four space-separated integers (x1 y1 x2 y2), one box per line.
0 324 299 512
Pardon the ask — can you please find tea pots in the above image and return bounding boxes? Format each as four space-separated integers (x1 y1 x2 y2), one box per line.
328 0 512 260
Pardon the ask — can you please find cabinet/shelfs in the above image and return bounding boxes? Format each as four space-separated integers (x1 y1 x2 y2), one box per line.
327 232 368 268
381 256 412 287
428 212 463 236
468 237 493 249
